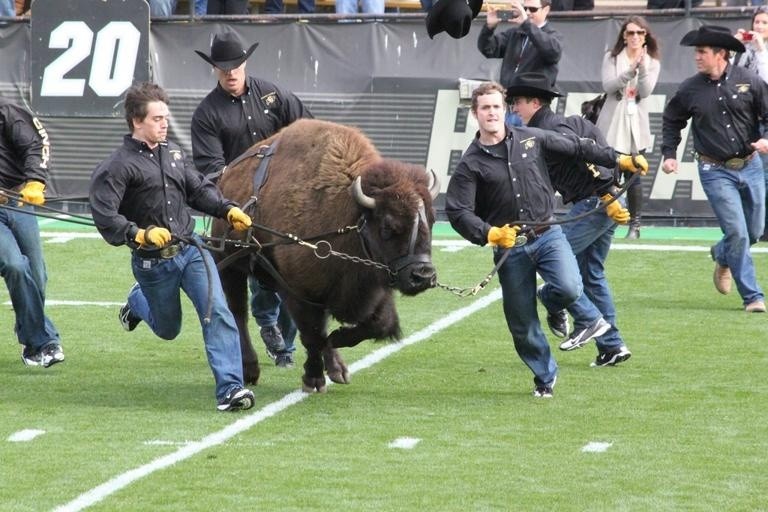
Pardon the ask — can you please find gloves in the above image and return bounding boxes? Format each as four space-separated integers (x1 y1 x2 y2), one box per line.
619 151 650 177
487 223 516 250
133 225 174 249
19 181 47 209
226 205 253 233
598 193 632 225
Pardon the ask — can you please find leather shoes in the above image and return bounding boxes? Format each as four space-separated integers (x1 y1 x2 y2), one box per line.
711 245 732 297
743 298 767 313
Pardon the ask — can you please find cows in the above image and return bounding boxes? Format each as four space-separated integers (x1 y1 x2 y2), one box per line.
211 118 440 393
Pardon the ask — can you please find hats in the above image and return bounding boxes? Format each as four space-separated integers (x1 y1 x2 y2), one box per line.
678 24 746 56
425 1 483 40
505 73 563 105
193 31 260 73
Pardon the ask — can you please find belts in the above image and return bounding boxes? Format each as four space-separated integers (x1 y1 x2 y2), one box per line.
695 151 758 171
570 186 617 205
512 217 552 247
130 234 194 259
0 181 26 207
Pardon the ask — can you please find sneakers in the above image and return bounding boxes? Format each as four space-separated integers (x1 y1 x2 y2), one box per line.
266 347 295 369
39 338 68 367
217 385 255 413
260 324 288 353
559 317 610 350
588 345 632 368
118 281 144 333
535 283 569 336
19 337 42 370
534 373 558 398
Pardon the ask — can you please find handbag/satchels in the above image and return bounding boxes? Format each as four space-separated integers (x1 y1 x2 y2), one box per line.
580 94 609 125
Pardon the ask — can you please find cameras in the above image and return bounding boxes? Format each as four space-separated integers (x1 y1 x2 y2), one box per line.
741 33 752 41
496 9 516 21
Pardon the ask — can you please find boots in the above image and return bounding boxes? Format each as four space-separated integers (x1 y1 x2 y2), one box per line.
625 182 643 242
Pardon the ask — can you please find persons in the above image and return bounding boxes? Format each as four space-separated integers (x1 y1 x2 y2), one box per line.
661 25 768 313
727 9 768 245
190 31 315 369
505 72 632 367
0 94 65 369
445 81 648 399
89 82 256 412
593 14 661 240
478 1 562 93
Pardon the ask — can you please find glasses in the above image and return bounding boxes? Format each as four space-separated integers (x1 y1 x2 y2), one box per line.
523 4 549 13
624 30 648 38
